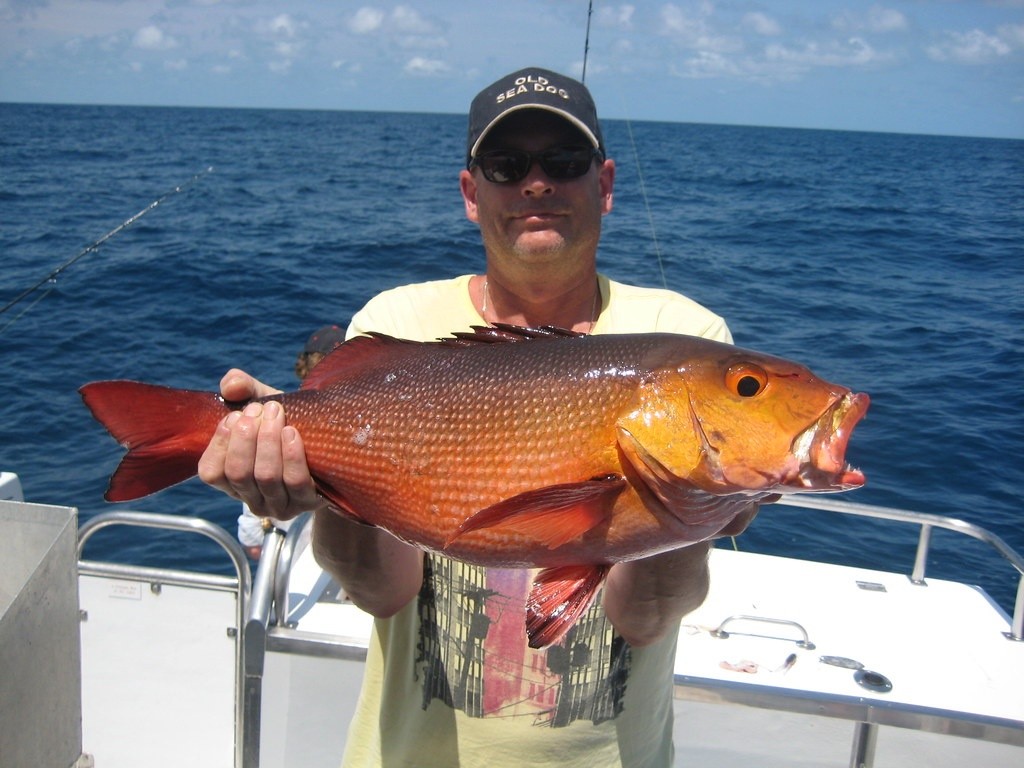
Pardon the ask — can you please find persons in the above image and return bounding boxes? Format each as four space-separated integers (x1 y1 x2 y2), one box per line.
236 325 350 605
198 66 786 768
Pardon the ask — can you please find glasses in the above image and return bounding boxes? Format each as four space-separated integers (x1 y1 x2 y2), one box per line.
468 139 605 184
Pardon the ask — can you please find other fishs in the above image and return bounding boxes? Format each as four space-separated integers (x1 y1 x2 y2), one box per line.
77 323 870 650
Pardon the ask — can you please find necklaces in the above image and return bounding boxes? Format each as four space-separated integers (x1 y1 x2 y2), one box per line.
476 271 602 337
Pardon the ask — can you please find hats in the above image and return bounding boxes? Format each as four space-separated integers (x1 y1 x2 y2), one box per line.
466 66 606 168
